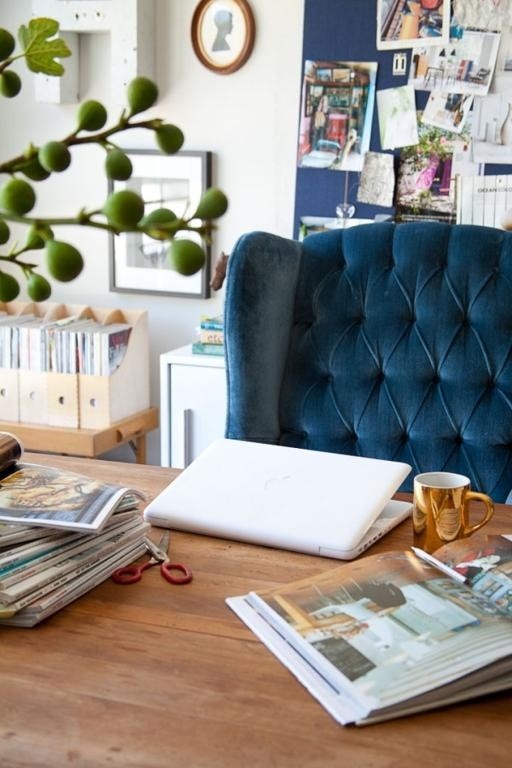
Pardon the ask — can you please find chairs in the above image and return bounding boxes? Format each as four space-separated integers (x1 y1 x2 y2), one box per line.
218 220 510 503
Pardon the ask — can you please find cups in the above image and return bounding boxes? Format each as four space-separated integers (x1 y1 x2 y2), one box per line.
413 472 496 554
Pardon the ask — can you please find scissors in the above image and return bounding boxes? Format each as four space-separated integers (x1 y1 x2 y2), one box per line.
112 528 192 585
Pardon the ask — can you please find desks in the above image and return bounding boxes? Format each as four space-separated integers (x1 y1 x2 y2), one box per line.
0 449 510 768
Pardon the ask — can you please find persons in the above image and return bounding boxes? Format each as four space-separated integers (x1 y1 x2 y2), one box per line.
313 96 331 153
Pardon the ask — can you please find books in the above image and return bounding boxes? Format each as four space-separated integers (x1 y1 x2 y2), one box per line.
0 308 132 376
0 433 151 630
226 528 512 726
193 315 227 356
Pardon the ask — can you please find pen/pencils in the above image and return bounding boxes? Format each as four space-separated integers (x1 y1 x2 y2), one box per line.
410 546 472 586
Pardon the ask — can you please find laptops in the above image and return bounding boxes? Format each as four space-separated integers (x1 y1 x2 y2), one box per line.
144 437 414 560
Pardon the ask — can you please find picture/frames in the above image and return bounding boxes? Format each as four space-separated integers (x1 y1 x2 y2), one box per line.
103 147 213 300
189 0 256 75
